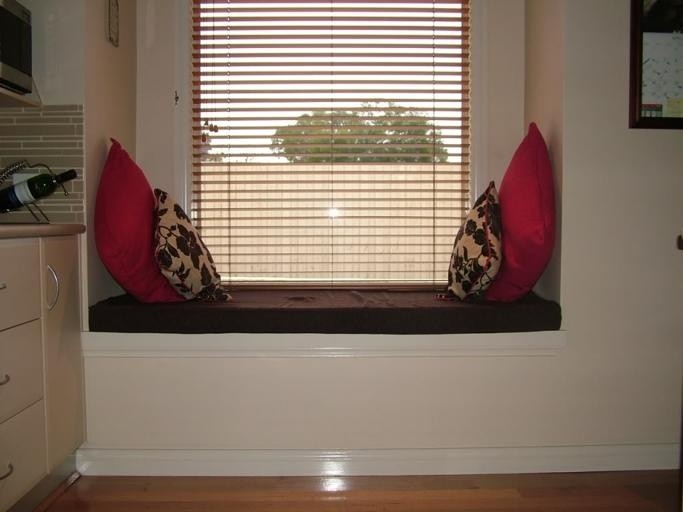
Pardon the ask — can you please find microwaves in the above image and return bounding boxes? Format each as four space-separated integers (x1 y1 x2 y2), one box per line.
0 0 32 95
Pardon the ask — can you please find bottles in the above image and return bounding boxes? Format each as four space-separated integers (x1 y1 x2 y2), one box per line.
0 169 77 212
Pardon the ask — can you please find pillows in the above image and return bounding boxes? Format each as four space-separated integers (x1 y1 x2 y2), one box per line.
436 120 556 304
94 136 234 304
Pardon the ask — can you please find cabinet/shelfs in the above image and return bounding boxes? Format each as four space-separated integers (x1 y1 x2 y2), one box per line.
0 223 87 512
